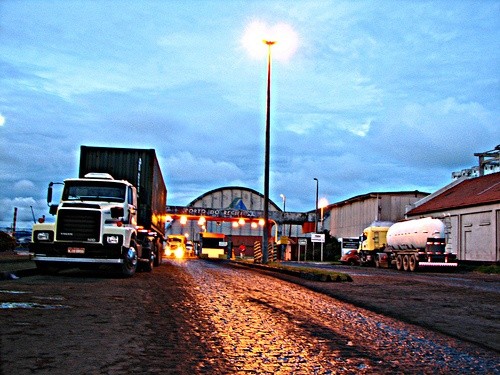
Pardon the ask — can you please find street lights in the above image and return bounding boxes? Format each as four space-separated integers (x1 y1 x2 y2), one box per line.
247 23 295 265
318 199 327 262
312 177 318 261
280 193 286 235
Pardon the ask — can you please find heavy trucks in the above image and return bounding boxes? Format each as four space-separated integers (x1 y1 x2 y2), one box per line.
27 144 168 277
358 217 457 272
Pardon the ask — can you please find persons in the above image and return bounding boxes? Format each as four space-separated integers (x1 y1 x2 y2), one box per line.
238 241 247 259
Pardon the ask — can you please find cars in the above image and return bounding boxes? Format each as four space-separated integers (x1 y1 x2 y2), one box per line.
337 250 359 266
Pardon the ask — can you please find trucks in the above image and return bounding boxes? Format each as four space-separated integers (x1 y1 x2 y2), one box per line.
165 235 195 260
